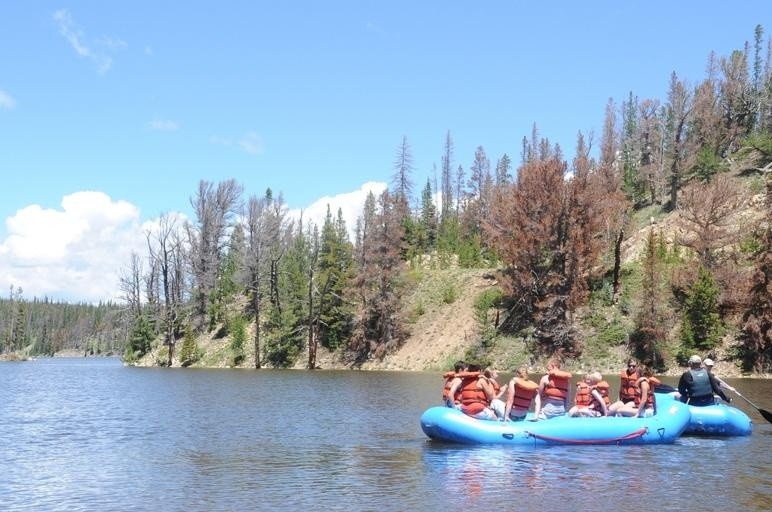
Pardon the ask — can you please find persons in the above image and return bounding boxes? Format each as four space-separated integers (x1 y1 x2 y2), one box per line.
700 358 735 393
607 359 644 415
442 360 468 410
674 355 732 405
484 366 508 407
489 365 541 421
616 364 654 418
527 360 572 420
447 441 734 497
448 363 499 421
575 373 593 407
569 372 610 418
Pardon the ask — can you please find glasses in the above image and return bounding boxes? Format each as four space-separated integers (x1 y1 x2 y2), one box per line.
628 365 635 367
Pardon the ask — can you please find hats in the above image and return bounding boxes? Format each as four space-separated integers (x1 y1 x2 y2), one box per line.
703 358 713 366
688 355 701 364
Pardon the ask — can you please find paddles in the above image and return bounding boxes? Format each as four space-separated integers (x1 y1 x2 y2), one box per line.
715 376 772 424
655 384 733 402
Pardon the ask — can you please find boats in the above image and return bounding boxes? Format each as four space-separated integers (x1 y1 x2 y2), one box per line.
673 390 752 436
420 397 692 445
